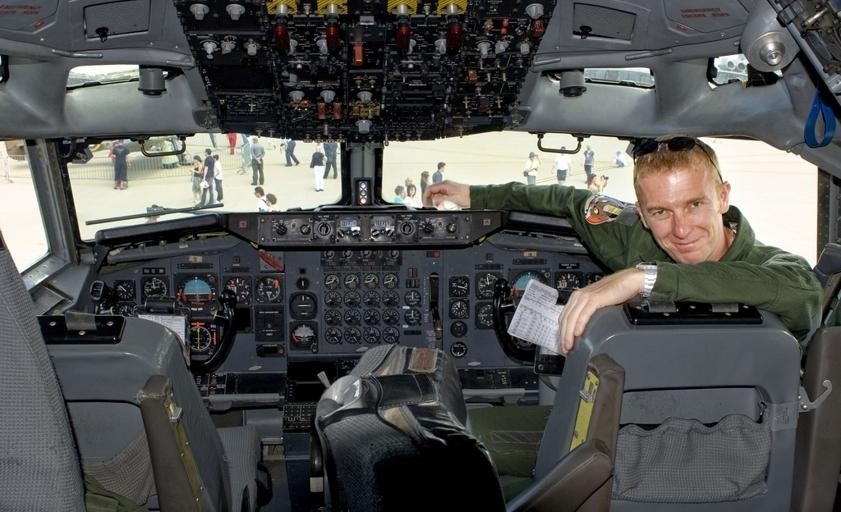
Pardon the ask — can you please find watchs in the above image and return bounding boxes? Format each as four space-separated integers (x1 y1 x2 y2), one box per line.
635 262 657 297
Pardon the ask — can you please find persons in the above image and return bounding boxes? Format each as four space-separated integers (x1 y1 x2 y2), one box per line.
420 133 823 354
110 142 121 186
583 145 596 177
394 162 445 208
585 173 599 193
109 140 130 189
551 146 572 184
615 151 624 168
525 152 538 184
190 132 337 212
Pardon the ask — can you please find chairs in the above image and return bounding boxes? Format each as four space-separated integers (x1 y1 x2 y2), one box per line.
38 309 262 512
510 297 841 512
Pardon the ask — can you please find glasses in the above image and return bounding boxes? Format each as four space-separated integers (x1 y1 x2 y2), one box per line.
630 135 696 158
